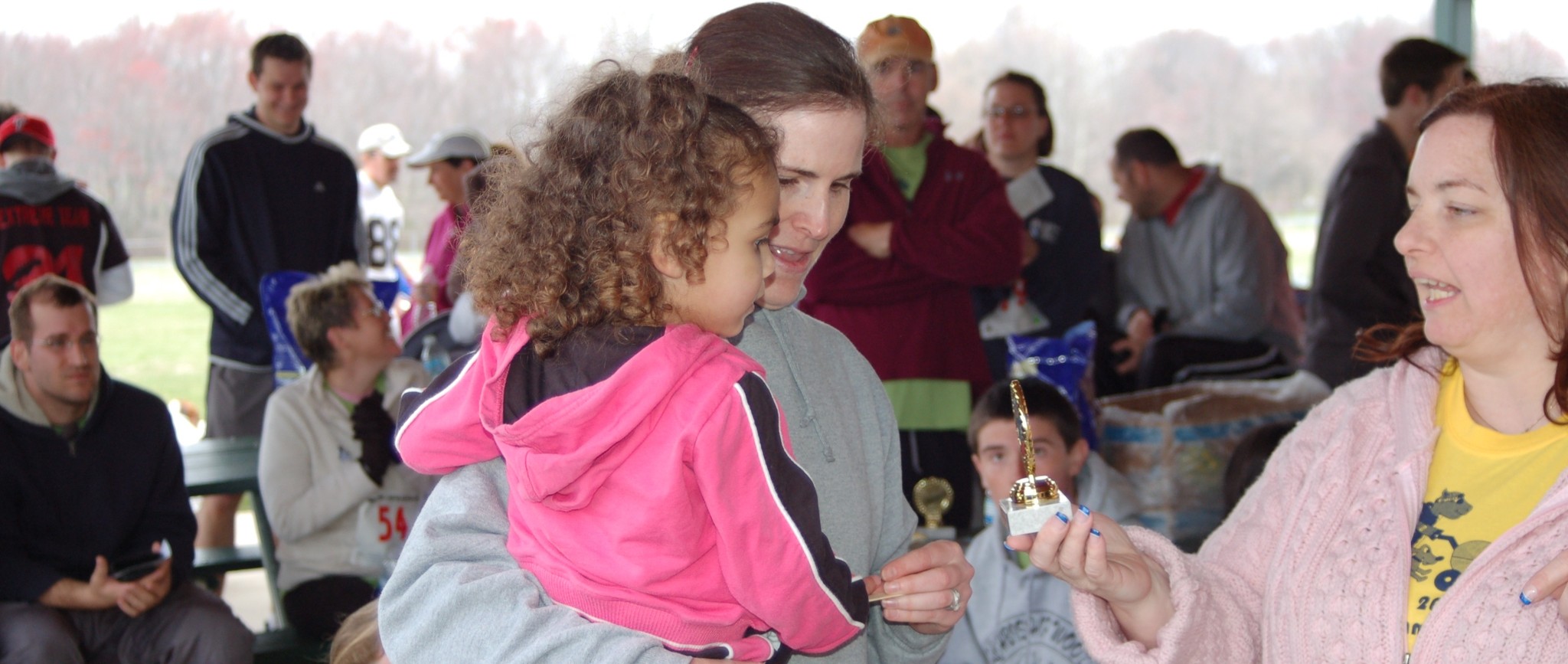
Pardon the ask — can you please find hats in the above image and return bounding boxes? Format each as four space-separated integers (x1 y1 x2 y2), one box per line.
356 123 411 158
0 114 55 149
853 13 934 66
407 130 490 169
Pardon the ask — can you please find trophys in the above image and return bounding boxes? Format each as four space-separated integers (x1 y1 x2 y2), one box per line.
913 476 956 540
998 380 1071 536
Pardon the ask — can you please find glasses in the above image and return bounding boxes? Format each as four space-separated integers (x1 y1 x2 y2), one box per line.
980 103 1038 119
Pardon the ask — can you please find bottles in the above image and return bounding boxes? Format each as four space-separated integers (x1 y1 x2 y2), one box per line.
420 336 452 378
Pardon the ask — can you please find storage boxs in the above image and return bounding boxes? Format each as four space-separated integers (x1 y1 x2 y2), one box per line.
1097 379 1308 542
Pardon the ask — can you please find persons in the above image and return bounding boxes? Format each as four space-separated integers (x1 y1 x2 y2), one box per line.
0 4 1568 664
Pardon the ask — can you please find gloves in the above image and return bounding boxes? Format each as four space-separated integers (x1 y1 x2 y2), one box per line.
350 388 404 485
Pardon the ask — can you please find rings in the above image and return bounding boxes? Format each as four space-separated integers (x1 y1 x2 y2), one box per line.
948 587 961 612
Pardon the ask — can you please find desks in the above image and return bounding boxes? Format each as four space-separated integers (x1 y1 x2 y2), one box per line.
168 436 286 627
